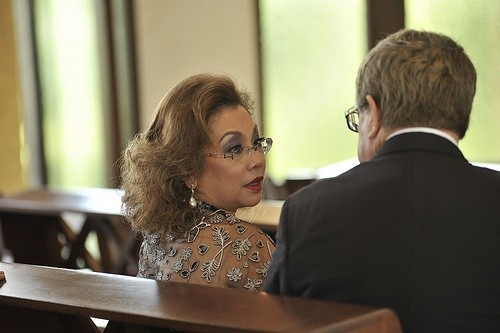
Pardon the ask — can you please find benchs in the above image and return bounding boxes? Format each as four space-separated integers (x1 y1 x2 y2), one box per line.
0 187 404 333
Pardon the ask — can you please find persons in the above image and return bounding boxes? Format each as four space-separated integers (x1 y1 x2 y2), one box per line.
262 29 500 333
121 74 277 290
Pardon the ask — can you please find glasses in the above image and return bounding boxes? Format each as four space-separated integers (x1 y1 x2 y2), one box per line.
345 98 368 132
200 137 273 164
